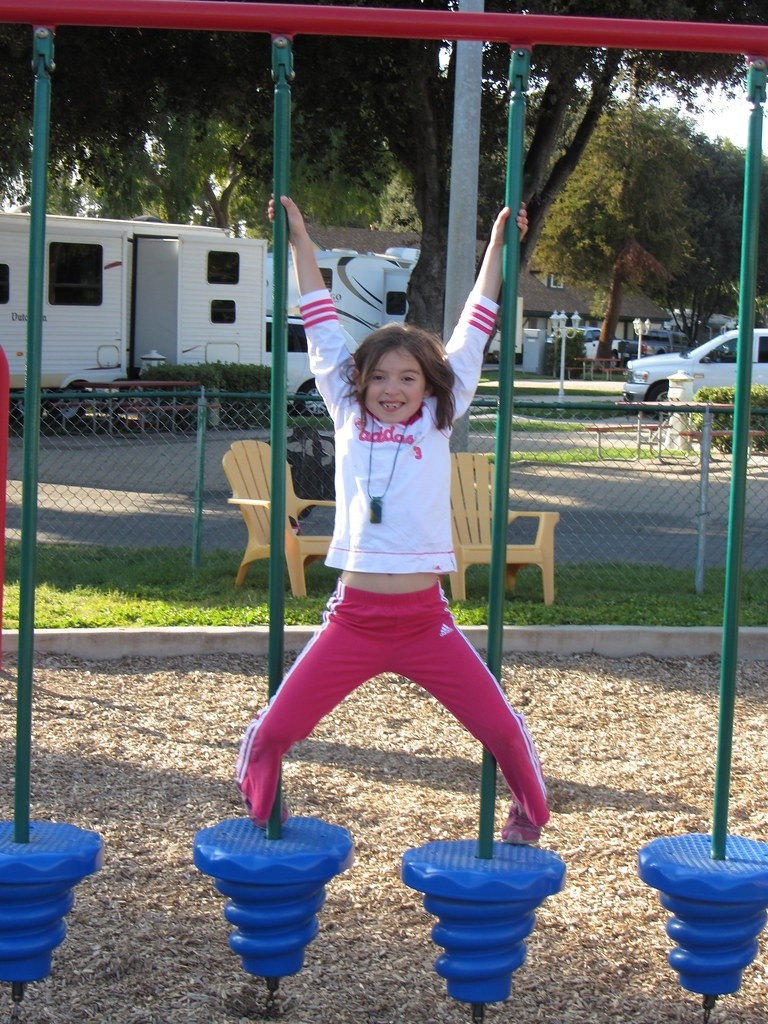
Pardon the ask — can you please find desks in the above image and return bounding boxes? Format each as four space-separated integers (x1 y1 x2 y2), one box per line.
616 401 734 467
574 358 621 380
72 381 198 439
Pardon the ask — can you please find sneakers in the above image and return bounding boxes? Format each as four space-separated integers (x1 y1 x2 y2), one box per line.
236 780 288 830
500 798 542 846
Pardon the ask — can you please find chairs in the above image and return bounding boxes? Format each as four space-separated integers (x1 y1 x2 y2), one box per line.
439 453 560 606
222 439 336 598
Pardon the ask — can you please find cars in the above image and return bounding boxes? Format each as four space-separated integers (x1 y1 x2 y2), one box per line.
622 327 767 420
546 327 623 368
266 315 332 417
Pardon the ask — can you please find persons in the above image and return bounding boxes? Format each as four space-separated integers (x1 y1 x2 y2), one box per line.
236 193 549 844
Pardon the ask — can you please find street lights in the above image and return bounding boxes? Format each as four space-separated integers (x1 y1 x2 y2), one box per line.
550 310 581 403
633 318 652 358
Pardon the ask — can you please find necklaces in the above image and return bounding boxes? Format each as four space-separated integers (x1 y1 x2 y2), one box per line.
367 417 412 523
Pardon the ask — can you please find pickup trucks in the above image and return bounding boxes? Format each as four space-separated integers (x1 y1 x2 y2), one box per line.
621 329 694 358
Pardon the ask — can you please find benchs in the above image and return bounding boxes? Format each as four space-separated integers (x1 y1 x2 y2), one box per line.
565 367 602 380
586 426 673 461
603 368 627 380
48 402 142 437
679 431 765 461
124 406 199 439
